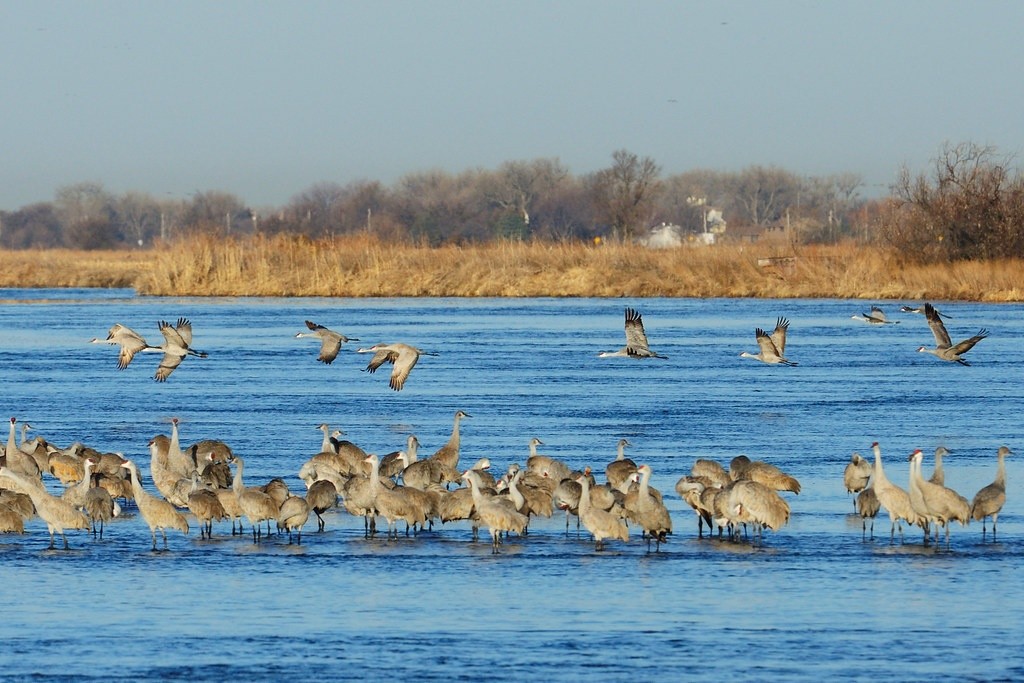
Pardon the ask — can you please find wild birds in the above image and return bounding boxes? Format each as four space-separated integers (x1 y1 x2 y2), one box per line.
365 343 441 391
599 307 670 360
294 319 362 364
851 306 900 327
899 304 953 319
915 302 991 367
741 316 800 368
88 316 209 382
0 416 1017 551
